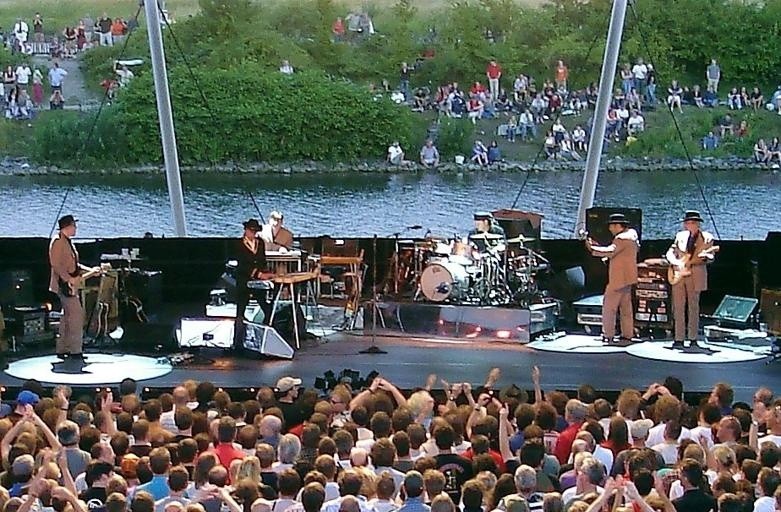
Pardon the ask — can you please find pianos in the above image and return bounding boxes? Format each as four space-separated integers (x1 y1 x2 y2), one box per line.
247 274 286 289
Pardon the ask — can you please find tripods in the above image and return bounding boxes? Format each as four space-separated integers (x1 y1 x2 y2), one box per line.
387 227 410 302
470 239 554 309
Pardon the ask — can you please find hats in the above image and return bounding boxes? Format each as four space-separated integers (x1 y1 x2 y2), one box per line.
609 213 630 224
631 419 654 439
243 219 262 231
277 376 302 392
18 390 38 405
57 215 79 230
474 213 490 220
681 210 704 221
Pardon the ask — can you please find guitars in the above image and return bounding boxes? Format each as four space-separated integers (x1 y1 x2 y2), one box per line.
63 263 112 296
667 245 720 285
578 230 610 266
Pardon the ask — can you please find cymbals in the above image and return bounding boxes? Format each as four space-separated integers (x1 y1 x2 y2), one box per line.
508 255 532 262
519 265 546 272
507 233 534 244
470 232 502 240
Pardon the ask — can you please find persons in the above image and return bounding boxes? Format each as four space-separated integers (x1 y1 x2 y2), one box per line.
603 60 781 167
0 2 179 120
231 218 278 354
332 5 374 44
261 213 295 250
1 369 781 512
465 211 508 248
279 60 293 75
583 209 642 344
367 26 601 167
48 215 103 360
666 209 714 349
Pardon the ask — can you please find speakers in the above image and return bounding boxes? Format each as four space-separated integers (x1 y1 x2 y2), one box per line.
243 320 294 359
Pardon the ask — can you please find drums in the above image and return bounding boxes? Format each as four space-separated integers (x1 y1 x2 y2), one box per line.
451 243 472 264
466 260 480 274
420 262 469 301
428 243 450 256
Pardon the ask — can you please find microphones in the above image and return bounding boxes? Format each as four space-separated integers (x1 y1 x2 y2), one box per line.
411 225 422 230
